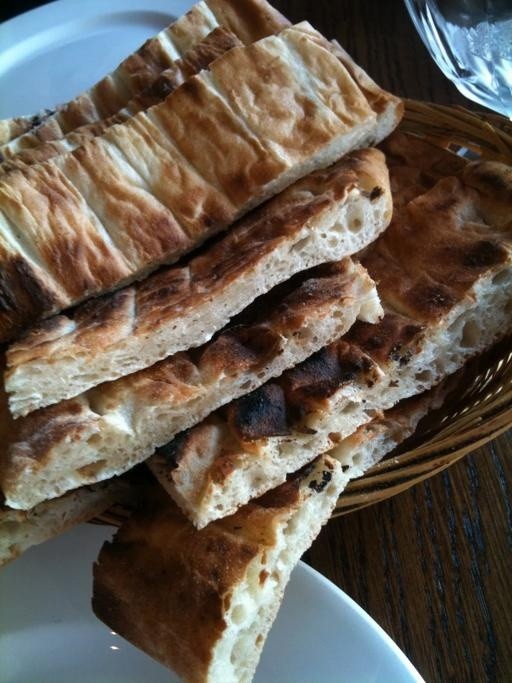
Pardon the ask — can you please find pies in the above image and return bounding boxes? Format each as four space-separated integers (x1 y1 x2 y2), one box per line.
1 2 511 683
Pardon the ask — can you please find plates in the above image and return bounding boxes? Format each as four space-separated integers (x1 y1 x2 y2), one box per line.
0 522 422 678
0 1 201 124
